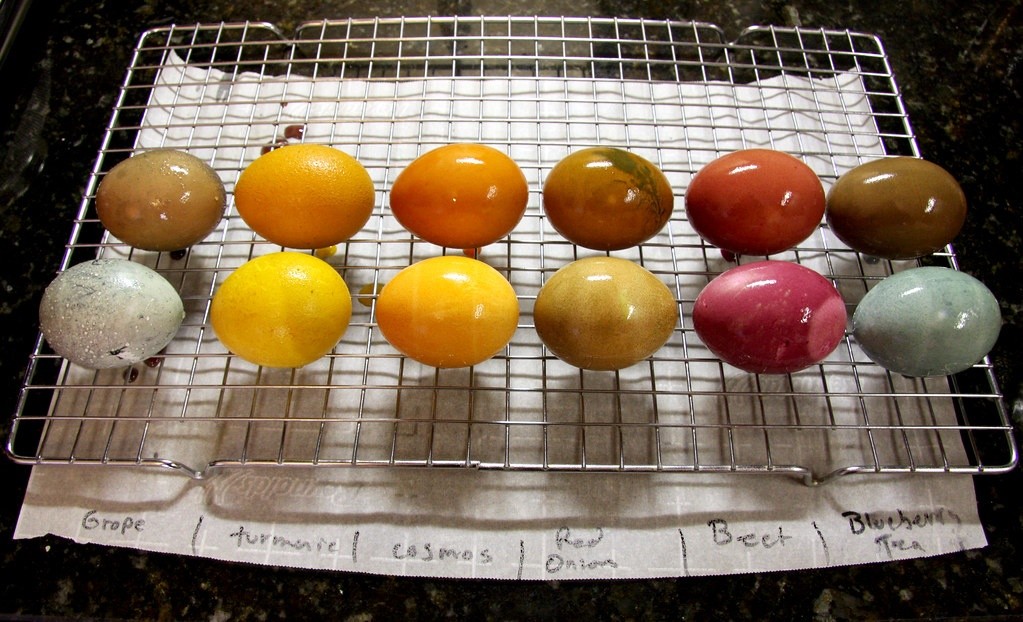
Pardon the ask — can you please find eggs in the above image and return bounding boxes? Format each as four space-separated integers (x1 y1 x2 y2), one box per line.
39 146 1002 378
